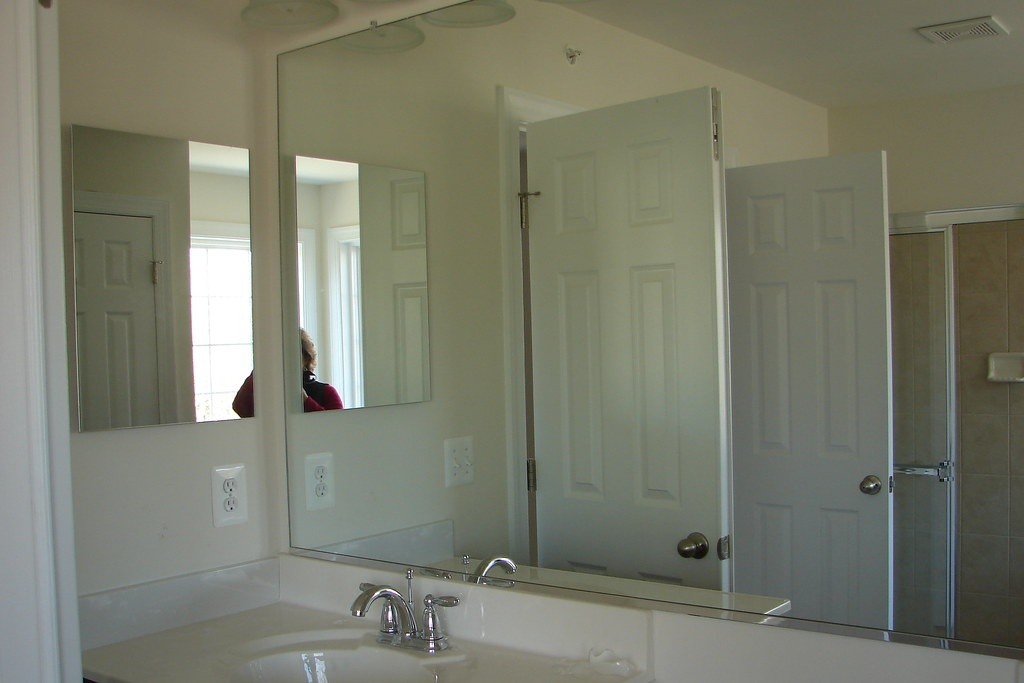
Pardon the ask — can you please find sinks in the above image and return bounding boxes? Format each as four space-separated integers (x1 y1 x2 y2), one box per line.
201 625 453 683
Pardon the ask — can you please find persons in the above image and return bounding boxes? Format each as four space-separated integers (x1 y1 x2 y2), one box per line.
232 329 343 419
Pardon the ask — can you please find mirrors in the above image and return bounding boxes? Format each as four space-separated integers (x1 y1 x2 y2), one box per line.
70 123 256 434
278 1 1024 662
294 154 432 414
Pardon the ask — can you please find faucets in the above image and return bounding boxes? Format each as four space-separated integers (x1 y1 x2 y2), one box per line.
465 554 519 584
350 582 417 636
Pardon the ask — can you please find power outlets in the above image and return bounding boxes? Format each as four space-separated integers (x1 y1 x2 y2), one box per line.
210 463 250 528
443 434 476 488
304 452 335 512
